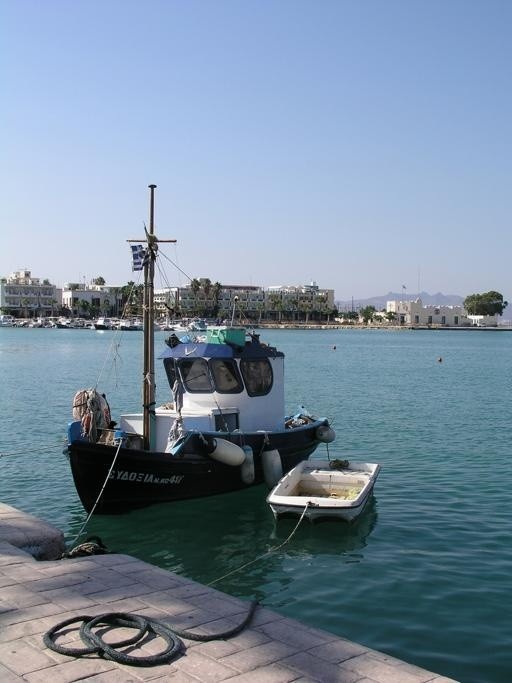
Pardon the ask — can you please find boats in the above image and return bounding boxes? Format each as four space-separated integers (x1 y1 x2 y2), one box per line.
62 183 330 515
1 314 208 331
265 458 382 524
